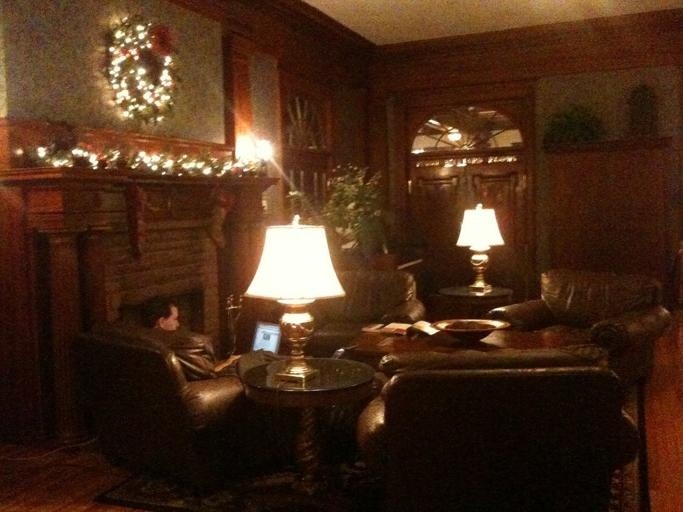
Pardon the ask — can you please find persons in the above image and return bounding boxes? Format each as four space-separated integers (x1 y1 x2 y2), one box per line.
141 295 242 380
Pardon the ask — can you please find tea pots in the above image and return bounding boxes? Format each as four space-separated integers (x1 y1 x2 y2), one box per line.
11 132 272 180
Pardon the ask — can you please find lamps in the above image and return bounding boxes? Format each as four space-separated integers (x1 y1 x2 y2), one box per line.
454 203 507 292
244 215 349 384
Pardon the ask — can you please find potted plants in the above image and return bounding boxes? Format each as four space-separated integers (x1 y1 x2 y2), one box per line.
318 160 402 272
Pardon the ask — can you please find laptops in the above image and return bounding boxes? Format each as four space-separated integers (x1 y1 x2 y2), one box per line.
250 321 282 355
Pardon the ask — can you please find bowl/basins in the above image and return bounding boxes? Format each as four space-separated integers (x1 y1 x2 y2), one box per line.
431 316 513 344
